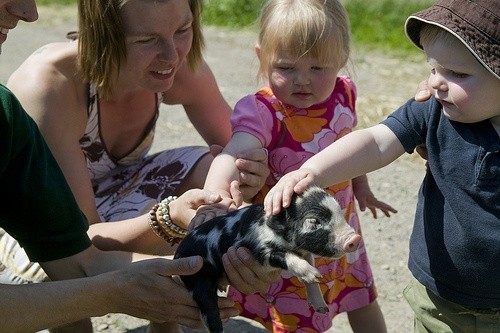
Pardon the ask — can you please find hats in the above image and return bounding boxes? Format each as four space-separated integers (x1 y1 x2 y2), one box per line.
404 0 500 78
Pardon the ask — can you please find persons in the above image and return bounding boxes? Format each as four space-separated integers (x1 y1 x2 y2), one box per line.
204 0 398 333
6 0 269 252
0 0 203 333
264 0 500 333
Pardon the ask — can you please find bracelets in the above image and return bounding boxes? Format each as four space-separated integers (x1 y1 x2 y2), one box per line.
155 203 181 238
162 196 194 235
150 204 172 243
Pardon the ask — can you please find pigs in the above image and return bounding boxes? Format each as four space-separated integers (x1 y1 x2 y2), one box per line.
172 183 361 333
